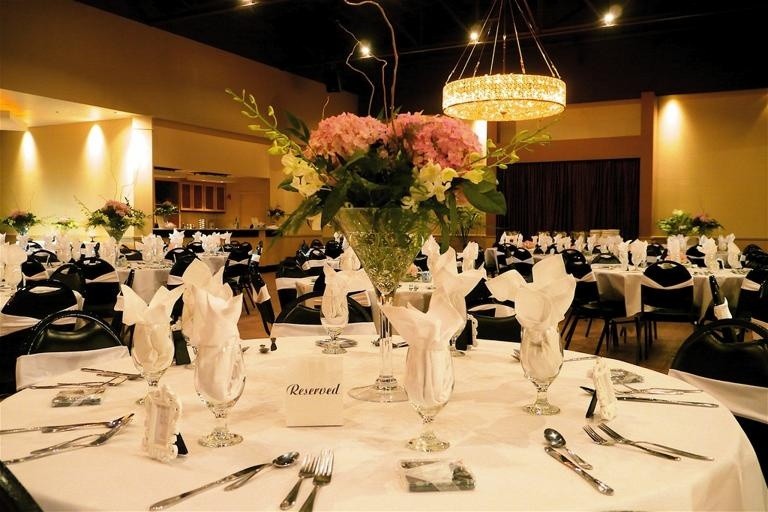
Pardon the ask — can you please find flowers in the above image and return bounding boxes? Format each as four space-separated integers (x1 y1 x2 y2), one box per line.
692 213 727 236
222 1 560 287
50 215 79 234
267 204 285 221
154 201 180 217
657 208 692 237
72 166 153 242
1 196 45 236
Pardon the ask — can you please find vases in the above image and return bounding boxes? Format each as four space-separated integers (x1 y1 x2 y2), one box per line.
102 223 129 266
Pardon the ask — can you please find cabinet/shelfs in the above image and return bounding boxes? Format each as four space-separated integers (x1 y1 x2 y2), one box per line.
153 229 282 273
153 176 232 228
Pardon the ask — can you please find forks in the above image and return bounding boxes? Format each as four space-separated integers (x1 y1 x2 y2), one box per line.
299 448 335 511
279 453 321 511
57 375 132 386
582 424 681 462
29 418 137 454
597 423 713 461
27 373 123 389
612 377 704 395
0 420 126 466
511 348 598 362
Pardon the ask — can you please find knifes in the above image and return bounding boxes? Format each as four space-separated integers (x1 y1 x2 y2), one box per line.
544 446 614 496
149 462 268 512
0 422 110 436
616 396 720 409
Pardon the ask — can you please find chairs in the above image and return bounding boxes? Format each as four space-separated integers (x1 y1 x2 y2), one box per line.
230 240 240 245
24 250 59 263
648 249 661 266
504 243 515 257
325 240 343 257
68 257 121 317
647 243 664 253
742 244 768 333
0 280 85 350
270 290 377 336
307 248 326 260
280 240 309 276
164 248 198 317
16 310 129 389
240 242 252 251
513 247 534 277
459 251 516 322
310 239 323 249
250 245 261 266
111 269 134 336
249 265 276 337
586 252 626 344
637 260 699 362
560 248 626 356
657 249 667 261
120 249 142 260
50 264 87 290
17 260 50 288
485 248 507 278
27 241 42 251
670 318 768 477
686 244 724 268
295 250 307 265
226 241 263 316
186 242 204 252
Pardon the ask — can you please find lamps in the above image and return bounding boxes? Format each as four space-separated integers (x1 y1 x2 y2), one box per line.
442 0 566 121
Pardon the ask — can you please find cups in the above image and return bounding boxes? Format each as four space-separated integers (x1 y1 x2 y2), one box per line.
449 301 467 357
320 295 349 354
131 324 174 405
404 349 456 452
193 344 246 447
520 329 564 416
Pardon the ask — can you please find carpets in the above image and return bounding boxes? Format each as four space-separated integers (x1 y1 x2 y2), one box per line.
237 272 752 373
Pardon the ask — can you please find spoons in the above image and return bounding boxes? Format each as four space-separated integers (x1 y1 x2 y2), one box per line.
223 451 301 492
580 384 596 396
97 372 141 380
543 427 594 470
259 344 269 354
41 413 135 434
